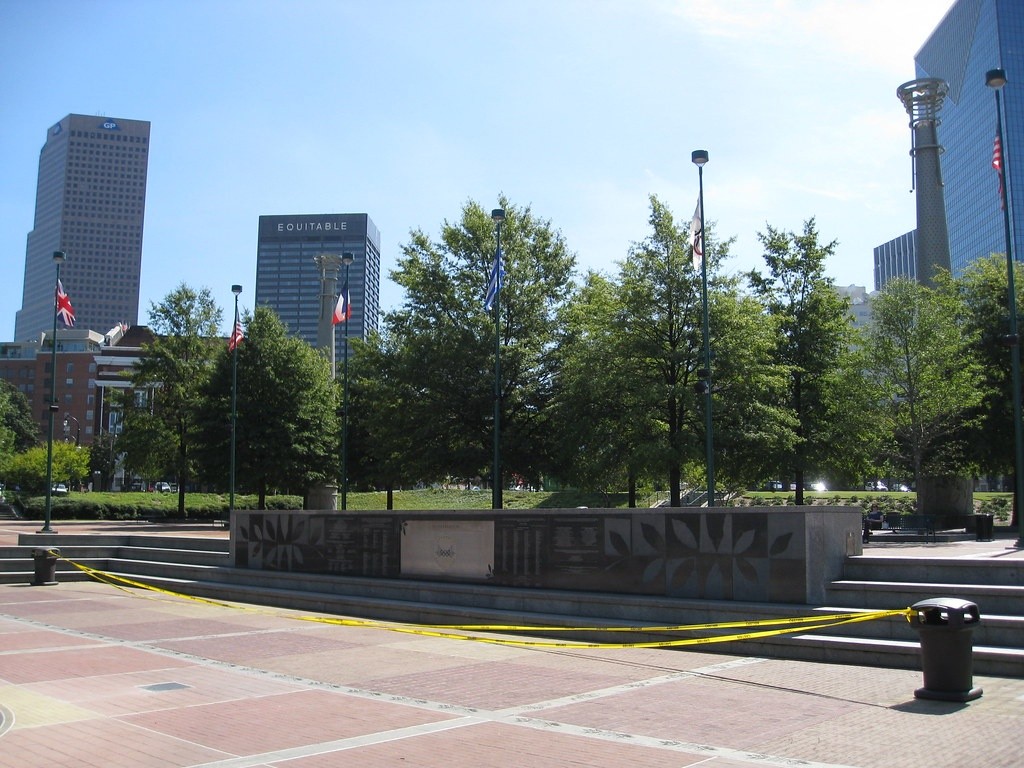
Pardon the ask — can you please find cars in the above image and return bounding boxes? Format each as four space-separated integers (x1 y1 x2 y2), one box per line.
891 483 912 491
809 482 828 491
791 482 806 491
169 483 180 494
52 484 68 497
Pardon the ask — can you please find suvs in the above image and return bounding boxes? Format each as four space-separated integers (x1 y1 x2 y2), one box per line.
765 481 783 491
156 481 171 493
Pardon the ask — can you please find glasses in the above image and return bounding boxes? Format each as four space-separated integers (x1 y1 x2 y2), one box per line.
873 506 877 508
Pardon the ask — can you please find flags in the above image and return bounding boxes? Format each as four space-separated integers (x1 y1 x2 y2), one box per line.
332 277 350 326
688 196 704 269
228 306 243 353
989 120 1006 210
55 279 75 327
486 245 506 313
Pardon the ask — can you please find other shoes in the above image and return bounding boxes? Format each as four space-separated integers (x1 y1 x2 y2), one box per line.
863 539 868 543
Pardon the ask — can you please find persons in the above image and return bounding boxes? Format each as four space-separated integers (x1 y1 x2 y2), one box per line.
77 482 85 493
141 481 146 492
88 482 93 493
120 481 126 492
157 482 161 491
866 504 884 529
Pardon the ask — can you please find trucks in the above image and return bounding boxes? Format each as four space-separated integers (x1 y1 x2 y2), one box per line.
442 475 480 490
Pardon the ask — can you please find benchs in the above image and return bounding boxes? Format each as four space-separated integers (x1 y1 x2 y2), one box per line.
861 514 937 544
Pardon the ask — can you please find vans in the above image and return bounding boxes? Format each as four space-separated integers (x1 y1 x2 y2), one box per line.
864 480 888 492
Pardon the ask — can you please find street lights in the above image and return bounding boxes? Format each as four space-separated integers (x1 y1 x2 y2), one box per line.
341 253 354 513
690 147 715 509
63 412 79 447
490 208 505 508
43 251 67 532
64 433 77 442
231 285 243 507
985 67 1024 546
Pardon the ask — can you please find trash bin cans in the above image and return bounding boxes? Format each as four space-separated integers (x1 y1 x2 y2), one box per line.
908 596 983 703
31 547 61 586
975 513 995 541
